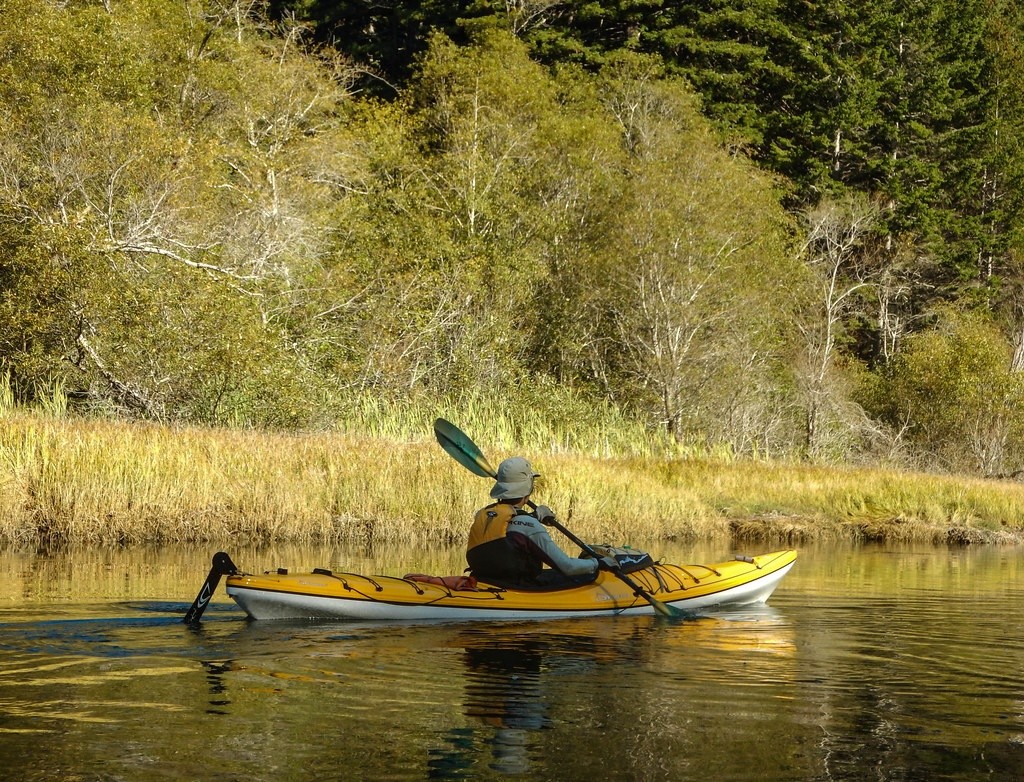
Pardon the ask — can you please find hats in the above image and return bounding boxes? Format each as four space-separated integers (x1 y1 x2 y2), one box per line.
490 457 532 499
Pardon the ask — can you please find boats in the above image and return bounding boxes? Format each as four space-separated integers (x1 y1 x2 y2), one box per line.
181 550 800 648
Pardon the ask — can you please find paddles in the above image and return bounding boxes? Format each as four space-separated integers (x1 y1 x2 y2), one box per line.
431 417 694 618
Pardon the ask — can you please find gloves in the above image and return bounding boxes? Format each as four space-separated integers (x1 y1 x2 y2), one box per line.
530 505 556 526
598 556 621 570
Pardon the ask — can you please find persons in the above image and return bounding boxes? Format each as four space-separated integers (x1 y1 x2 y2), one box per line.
465 457 620 589
